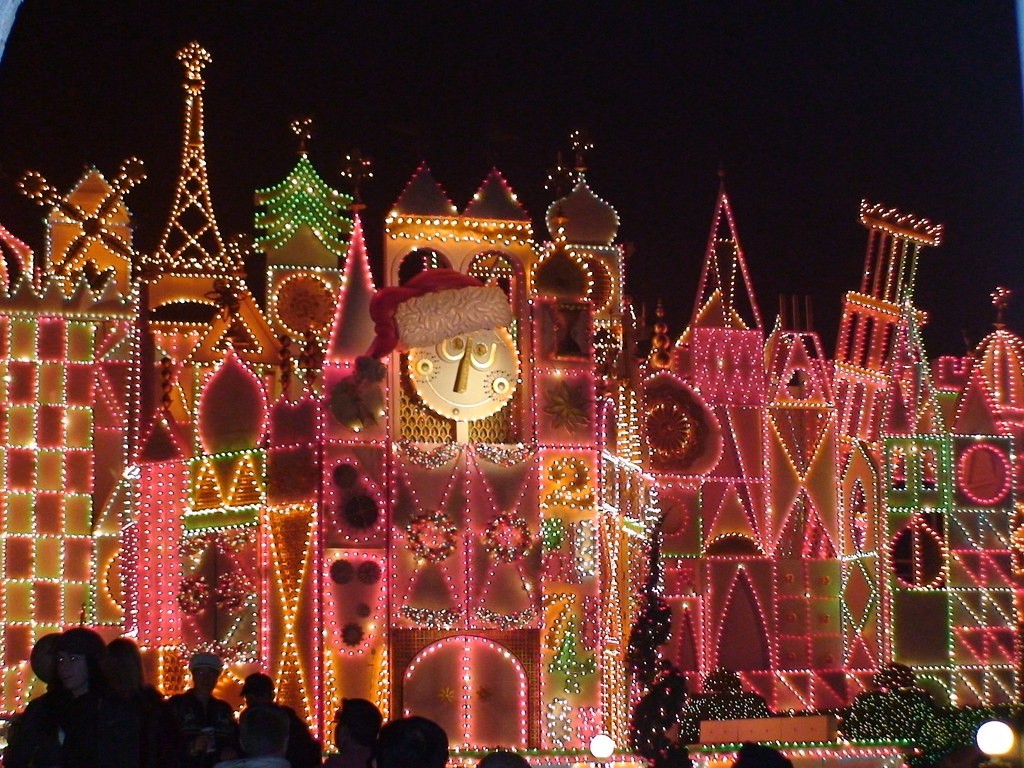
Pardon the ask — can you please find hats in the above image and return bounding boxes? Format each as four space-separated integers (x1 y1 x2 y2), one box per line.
49 627 106 660
189 652 223 671
240 673 275 696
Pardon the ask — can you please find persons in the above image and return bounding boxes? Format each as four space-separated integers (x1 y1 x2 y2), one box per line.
0 627 530 768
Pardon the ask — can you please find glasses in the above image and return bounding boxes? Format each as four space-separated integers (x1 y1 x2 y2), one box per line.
193 670 218 677
